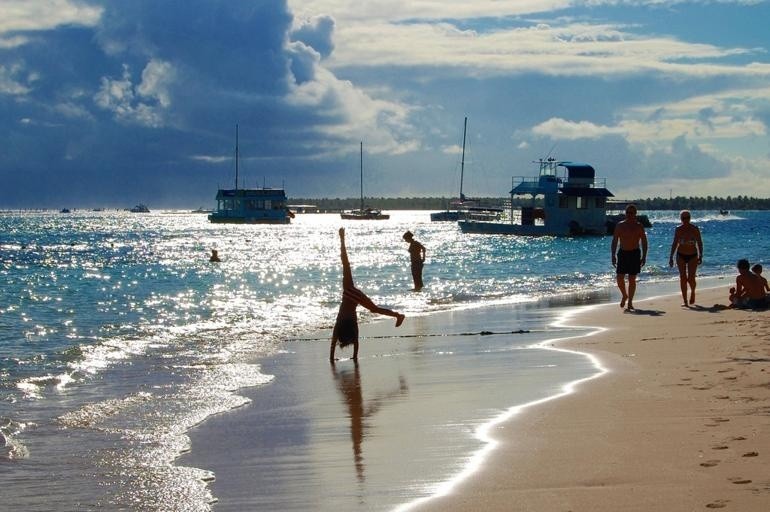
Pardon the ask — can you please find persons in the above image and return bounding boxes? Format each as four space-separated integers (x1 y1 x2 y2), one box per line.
611 204 647 309
329 226 406 361
740 263 769 294
727 258 765 309
209 249 222 262
668 210 703 307
402 230 426 291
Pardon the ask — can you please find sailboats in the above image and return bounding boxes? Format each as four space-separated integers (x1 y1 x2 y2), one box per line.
429 114 501 221
338 140 389 221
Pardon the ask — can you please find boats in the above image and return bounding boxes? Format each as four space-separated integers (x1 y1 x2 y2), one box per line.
208 122 294 225
458 158 651 236
130 203 150 212
